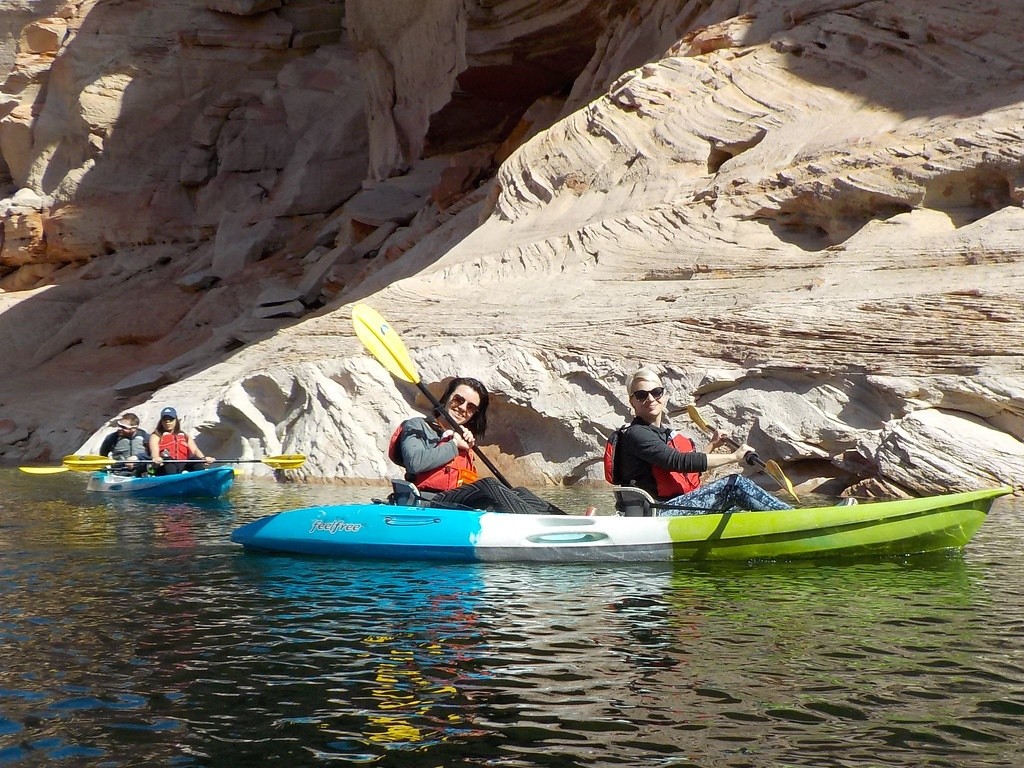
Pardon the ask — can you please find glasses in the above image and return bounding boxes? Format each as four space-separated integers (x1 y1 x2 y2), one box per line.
117 421 137 430
450 394 479 414
630 387 665 402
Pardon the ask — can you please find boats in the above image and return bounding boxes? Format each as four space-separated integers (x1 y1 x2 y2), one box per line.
229 479 1014 566
86 466 236 500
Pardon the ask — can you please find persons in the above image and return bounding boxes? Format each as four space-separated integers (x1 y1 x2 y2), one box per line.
148 407 216 477
388 377 595 515
99 412 151 477
604 369 858 516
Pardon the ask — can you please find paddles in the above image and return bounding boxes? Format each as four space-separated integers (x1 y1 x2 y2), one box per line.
686 406 800 502
351 303 514 491
18 464 242 476
63 455 307 468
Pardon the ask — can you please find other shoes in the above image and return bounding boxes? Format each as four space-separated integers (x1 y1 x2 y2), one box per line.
583 507 596 517
836 497 857 506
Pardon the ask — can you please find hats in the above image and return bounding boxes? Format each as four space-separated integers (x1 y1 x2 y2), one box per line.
161 407 176 419
